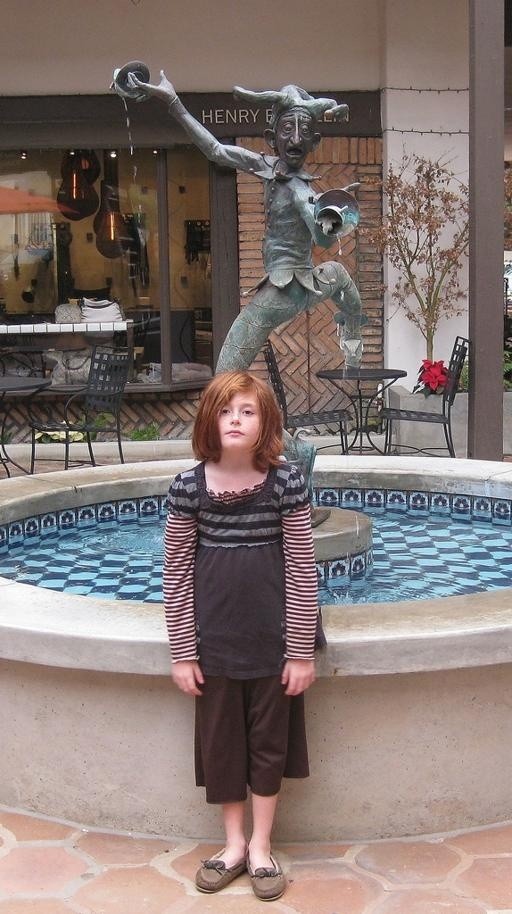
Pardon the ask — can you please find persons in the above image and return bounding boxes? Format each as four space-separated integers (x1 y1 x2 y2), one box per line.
126 60 368 518
158 376 318 902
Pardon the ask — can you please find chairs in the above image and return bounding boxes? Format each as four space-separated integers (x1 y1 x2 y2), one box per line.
255 339 351 455
377 335 467 458
27 342 137 475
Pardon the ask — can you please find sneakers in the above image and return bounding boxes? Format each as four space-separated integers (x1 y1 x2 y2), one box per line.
195 845 248 893
246 848 286 901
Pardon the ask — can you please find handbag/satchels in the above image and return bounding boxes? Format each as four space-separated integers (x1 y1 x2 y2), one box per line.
43 342 117 386
54 304 80 323
79 297 123 322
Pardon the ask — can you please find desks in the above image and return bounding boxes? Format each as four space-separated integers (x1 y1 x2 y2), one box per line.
314 364 406 455
0 374 54 480
